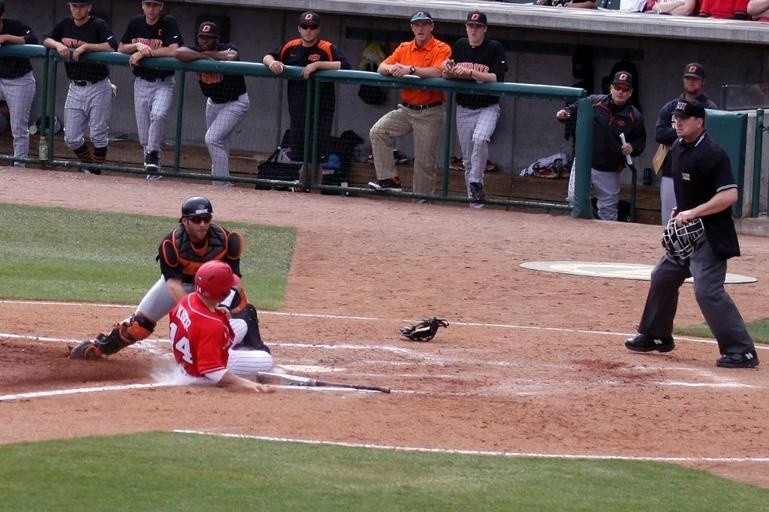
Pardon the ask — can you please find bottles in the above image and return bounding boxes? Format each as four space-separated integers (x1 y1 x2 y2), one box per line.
38 135 49 166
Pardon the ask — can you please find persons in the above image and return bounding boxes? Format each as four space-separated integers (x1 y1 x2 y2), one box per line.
655 62 720 231
174 22 252 190
622 96 762 369
443 11 507 212
0 2 43 165
262 9 353 156
117 2 183 183
42 1 119 175
66 195 271 359
363 7 454 203
533 0 768 26
555 70 650 223
169 261 286 395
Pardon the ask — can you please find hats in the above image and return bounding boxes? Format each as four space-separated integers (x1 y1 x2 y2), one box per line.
196 21 220 38
408 10 434 23
670 98 705 118
464 10 487 28
297 10 320 25
612 71 633 87
682 63 705 80
142 0 162 5
69 0 90 5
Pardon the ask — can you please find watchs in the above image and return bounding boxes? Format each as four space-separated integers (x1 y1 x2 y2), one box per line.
410 64 417 74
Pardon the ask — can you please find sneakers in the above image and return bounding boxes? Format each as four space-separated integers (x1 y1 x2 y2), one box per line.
143 150 160 169
68 338 94 360
367 148 410 166
625 330 675 353
368 175 403 193
468 182 485 202
146 174 162 181
448 157 466 171
557 166 570 178
484 163 496 172
532 164 559 179
716 348 760 367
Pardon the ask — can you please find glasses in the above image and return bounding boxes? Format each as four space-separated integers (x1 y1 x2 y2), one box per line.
299 24 319 30
612 84 630 92
184 214 213 224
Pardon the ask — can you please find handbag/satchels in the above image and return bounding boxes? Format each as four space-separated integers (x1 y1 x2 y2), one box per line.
254 143 304 191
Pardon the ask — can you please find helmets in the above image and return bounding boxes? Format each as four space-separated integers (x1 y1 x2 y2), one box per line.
181 195 214 217
35 115 62 135
193 259 241 301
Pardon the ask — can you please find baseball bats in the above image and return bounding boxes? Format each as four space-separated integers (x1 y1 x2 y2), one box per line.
256 372 390 394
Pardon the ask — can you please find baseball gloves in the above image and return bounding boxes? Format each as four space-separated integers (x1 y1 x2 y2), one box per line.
400 317 449 342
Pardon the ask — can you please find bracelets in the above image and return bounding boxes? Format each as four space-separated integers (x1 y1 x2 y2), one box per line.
468 69 474 78
267 58 279 70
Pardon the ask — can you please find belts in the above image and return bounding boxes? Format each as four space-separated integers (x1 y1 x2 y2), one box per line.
210 97 239 104
74 80 97 86
140 75 165 82
402 100 442 110
